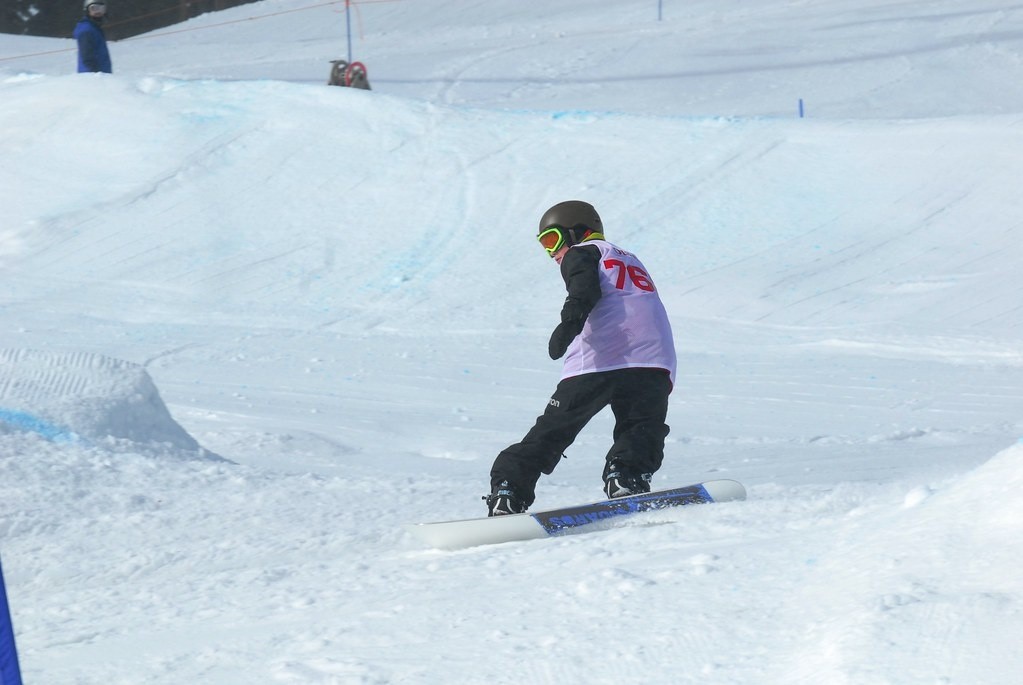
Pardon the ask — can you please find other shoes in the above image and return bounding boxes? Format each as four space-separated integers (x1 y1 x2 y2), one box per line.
606 465 648 498
488 481 527 516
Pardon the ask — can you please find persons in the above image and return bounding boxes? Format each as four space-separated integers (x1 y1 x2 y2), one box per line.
72 0 112 74
482 200 677 518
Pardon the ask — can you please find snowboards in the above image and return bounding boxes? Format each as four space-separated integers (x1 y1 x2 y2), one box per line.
408 479 750 551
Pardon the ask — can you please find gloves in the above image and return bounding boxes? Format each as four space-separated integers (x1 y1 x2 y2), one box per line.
549 321 580 360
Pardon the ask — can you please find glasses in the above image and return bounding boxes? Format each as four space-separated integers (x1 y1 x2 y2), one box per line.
538 228 564 258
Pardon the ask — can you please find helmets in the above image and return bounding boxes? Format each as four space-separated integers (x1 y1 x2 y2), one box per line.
539 201 604 235
